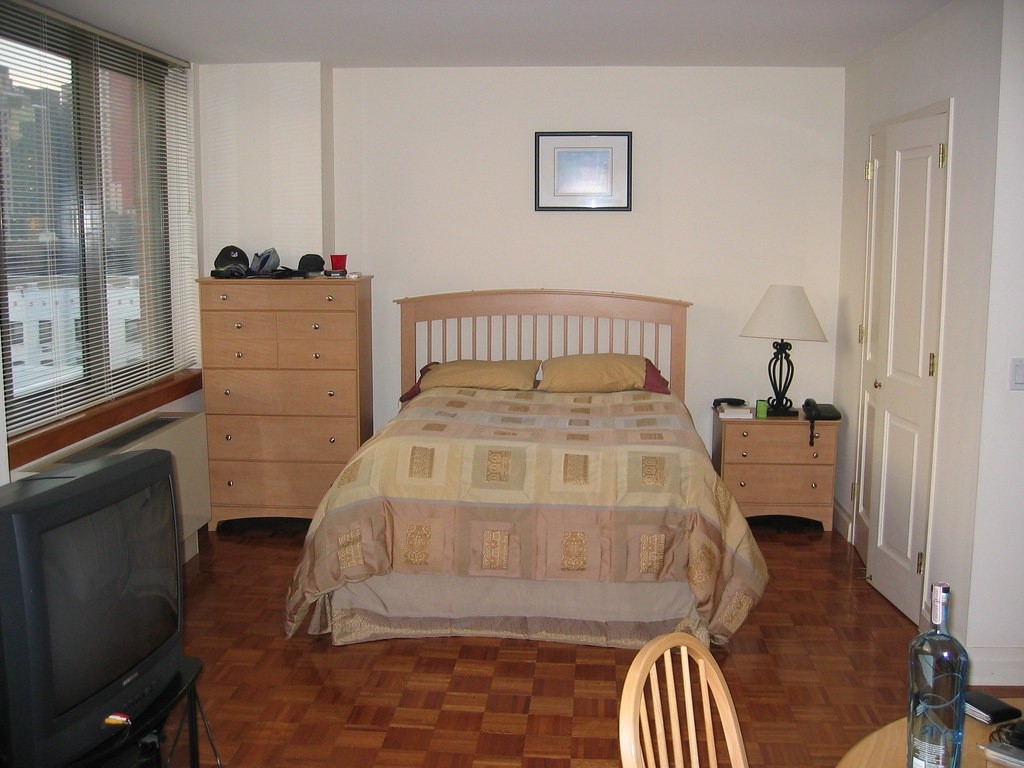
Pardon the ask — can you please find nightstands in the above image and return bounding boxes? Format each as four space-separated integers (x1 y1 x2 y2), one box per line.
709 405 841 532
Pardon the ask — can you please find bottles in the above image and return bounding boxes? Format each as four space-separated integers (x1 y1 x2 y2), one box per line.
907 582 969 768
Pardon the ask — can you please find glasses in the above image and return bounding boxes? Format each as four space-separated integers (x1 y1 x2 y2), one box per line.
271 266 306 279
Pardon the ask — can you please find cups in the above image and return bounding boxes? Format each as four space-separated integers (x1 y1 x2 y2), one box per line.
330 254 347 270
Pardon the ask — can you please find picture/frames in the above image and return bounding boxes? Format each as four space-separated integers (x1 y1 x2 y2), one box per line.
534 131 632 213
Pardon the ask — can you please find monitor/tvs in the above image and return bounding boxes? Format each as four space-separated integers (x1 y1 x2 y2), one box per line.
0 447 183 768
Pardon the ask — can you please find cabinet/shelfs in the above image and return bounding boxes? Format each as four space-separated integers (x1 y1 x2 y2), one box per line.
196 275 374 533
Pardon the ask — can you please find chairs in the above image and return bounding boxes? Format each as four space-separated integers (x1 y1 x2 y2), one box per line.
619 630 750 768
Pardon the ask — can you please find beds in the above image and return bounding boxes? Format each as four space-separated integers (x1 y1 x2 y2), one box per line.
285 282 770 654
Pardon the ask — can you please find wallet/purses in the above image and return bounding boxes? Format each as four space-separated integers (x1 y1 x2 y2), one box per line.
964 690 1021 724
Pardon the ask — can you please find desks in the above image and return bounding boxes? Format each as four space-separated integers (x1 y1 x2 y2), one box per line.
836 698 1024 768
75 656 204 768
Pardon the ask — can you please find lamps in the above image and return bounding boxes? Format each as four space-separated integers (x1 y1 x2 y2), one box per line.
739 285 827 417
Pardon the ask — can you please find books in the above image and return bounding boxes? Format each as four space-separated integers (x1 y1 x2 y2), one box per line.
964 692 1021 725
716 402 754 419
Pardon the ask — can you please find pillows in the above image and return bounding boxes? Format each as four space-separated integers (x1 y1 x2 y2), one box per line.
537 353 670 393
400 358 542 394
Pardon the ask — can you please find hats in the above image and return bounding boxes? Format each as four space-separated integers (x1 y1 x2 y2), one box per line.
215 246 249 274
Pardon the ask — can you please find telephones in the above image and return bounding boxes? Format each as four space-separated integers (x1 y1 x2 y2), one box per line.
802 398 842 421
